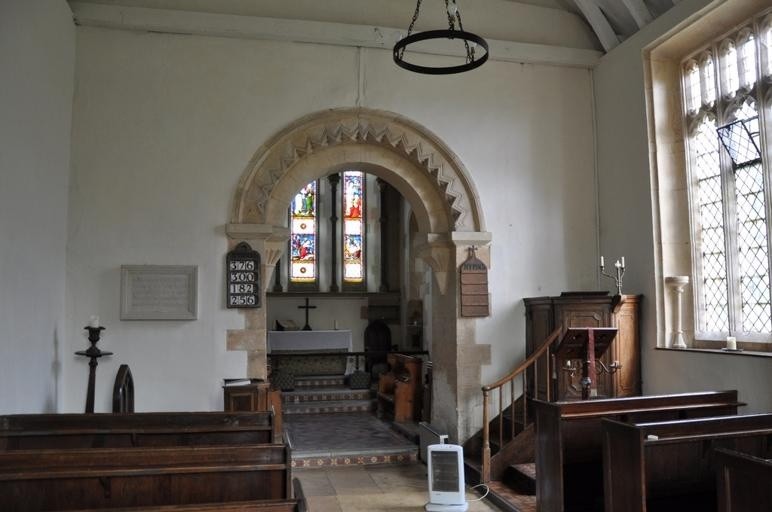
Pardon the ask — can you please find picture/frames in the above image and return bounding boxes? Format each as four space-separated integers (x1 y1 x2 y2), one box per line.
119 263 199 320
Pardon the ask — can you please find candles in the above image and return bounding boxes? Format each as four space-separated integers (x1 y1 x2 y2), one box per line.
334 320 338 329
615 260 621 266
601 255 604 266
89 314 100 328
621 257 625 268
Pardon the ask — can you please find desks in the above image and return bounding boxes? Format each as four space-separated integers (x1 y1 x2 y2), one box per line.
267 329 353 376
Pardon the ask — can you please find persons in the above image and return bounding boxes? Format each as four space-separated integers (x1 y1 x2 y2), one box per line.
364 308 391 377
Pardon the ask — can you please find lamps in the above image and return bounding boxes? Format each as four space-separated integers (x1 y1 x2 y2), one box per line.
391 0 489 75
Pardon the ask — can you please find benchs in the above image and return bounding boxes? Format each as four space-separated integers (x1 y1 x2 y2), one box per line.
158 478 307 512
3 412 275 450
533 388 772 511
3 431 295 508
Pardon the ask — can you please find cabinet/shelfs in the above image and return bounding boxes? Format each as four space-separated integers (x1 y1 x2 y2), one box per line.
223 382 269 410
520 292 642 398
